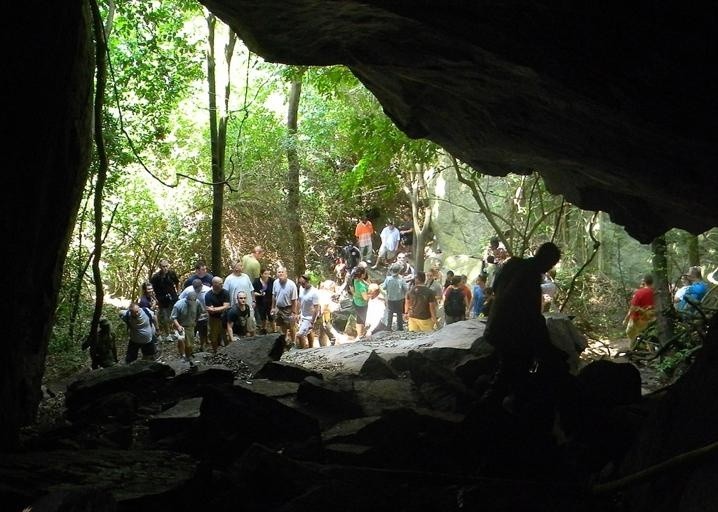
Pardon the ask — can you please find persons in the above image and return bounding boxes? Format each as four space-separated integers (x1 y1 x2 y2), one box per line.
139 282 158 312
473 242 564 412
541 268 557 298
672 266 705 310
120 304 160 363
171 245 340 358
468 239 506 317
624 274 657 345
87 319 117 369
333 215 472 339
149 259 180 342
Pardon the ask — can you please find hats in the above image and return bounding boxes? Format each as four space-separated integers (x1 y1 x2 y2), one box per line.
392 263 401 276
99 319 110 329
232 259 240 269
